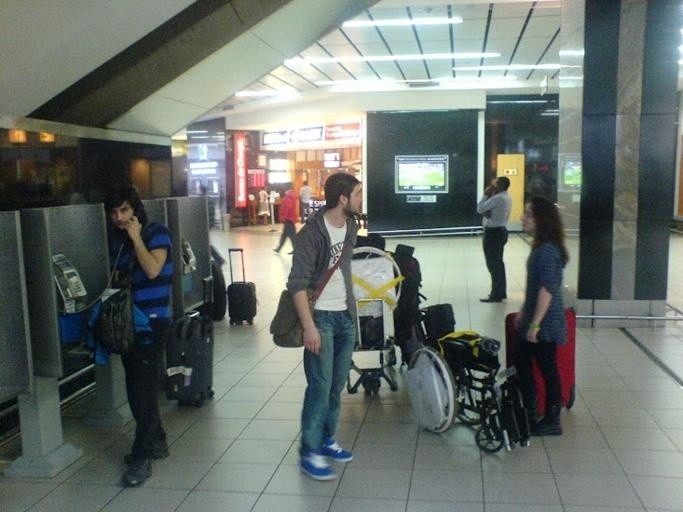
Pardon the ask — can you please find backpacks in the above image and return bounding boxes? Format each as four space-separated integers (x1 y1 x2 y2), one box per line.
91 268 138 358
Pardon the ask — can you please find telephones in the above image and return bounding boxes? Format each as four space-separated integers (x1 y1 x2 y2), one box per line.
120 204 142 242
182 235 196 275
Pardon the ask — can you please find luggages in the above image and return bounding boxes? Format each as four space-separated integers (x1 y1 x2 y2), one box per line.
164 308 215 409
504 304 578 420
227 280 257 326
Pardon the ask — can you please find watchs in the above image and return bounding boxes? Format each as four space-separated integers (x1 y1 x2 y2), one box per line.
527 323 541 330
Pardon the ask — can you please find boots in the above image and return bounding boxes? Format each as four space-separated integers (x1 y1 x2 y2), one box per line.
529 400 566 438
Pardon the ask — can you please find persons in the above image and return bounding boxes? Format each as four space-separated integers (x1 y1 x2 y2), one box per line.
198 184 208 196
514 198 569 435
257 187 270 224
270 173 363 480
272 187 299 255
90 185 173 487
300 180 312 224
477 176 512 302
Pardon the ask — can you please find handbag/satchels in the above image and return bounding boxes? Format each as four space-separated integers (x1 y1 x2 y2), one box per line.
268 286 317 350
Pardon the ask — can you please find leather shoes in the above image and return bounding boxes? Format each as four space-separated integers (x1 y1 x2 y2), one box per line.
479 295 502 303
488 294 508 300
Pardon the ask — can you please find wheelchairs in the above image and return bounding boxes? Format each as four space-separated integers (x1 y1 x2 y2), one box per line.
406 302 531 454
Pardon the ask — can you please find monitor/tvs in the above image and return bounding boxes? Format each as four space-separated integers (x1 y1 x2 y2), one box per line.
393 154 451 197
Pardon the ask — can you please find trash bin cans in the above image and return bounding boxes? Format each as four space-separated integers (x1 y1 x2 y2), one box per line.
222 214 230 230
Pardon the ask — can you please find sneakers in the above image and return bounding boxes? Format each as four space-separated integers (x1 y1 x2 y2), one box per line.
321 441 354 464
121 457 154 486
299 446 339 483
123 452 169 465
